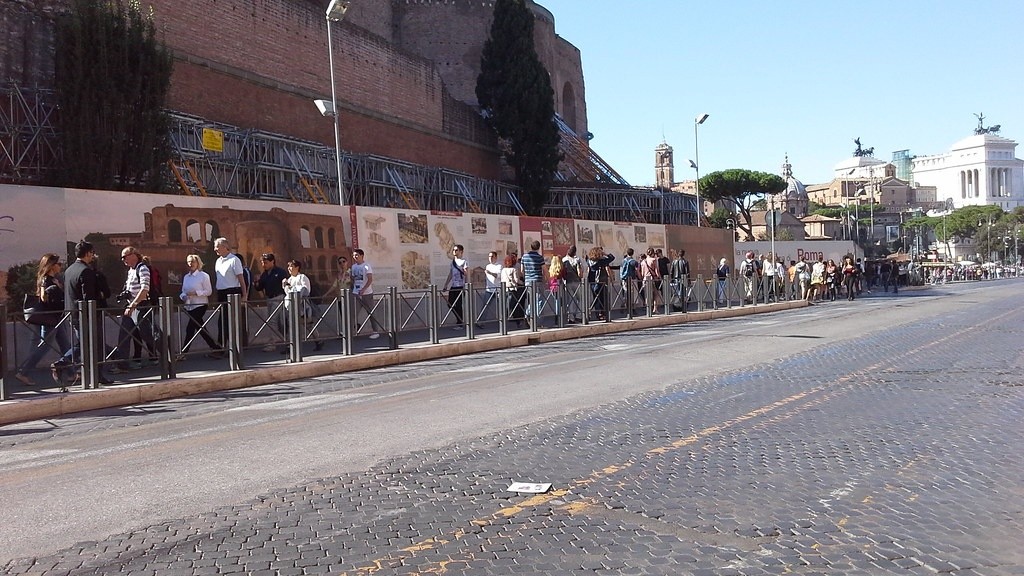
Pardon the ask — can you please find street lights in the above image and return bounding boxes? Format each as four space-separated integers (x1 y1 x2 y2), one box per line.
313 0 353 207
652 149 672 225
838 168 856 241
688 113 710 227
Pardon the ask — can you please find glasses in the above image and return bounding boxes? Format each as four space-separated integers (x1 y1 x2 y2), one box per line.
54 262 62 267
452 249 458 252
339 260 347 265
90 251 95 255
120 252 134 261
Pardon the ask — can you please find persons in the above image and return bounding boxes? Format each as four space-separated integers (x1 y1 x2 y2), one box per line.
739 251 908 304
336 248 379 339
977 268 982 281
716 258 730 304
443 244 470 330
15 242 159 384
984 268 988 279
176 238 325 360
475 241 615 329
619 248 691 314
925 267 952 283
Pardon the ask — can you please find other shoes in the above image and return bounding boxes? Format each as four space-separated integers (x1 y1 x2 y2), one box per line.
280 348 289 355
51 360 61 384
263 345 275 351
98 375 114 385
454 305 682 331
314 340 324 352
369 334 380 339
68 374 82 381
176 354 187 360
109 366 130 374
209 351 226 358
16 373 36 386
133 363 142 370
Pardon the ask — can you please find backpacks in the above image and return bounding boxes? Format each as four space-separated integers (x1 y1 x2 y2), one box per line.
745 259 754 277
135 263 161 303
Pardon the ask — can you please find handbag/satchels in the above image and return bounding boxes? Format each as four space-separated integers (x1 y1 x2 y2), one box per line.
24 281 58 325
655 281 663 291
596 268 607 283
